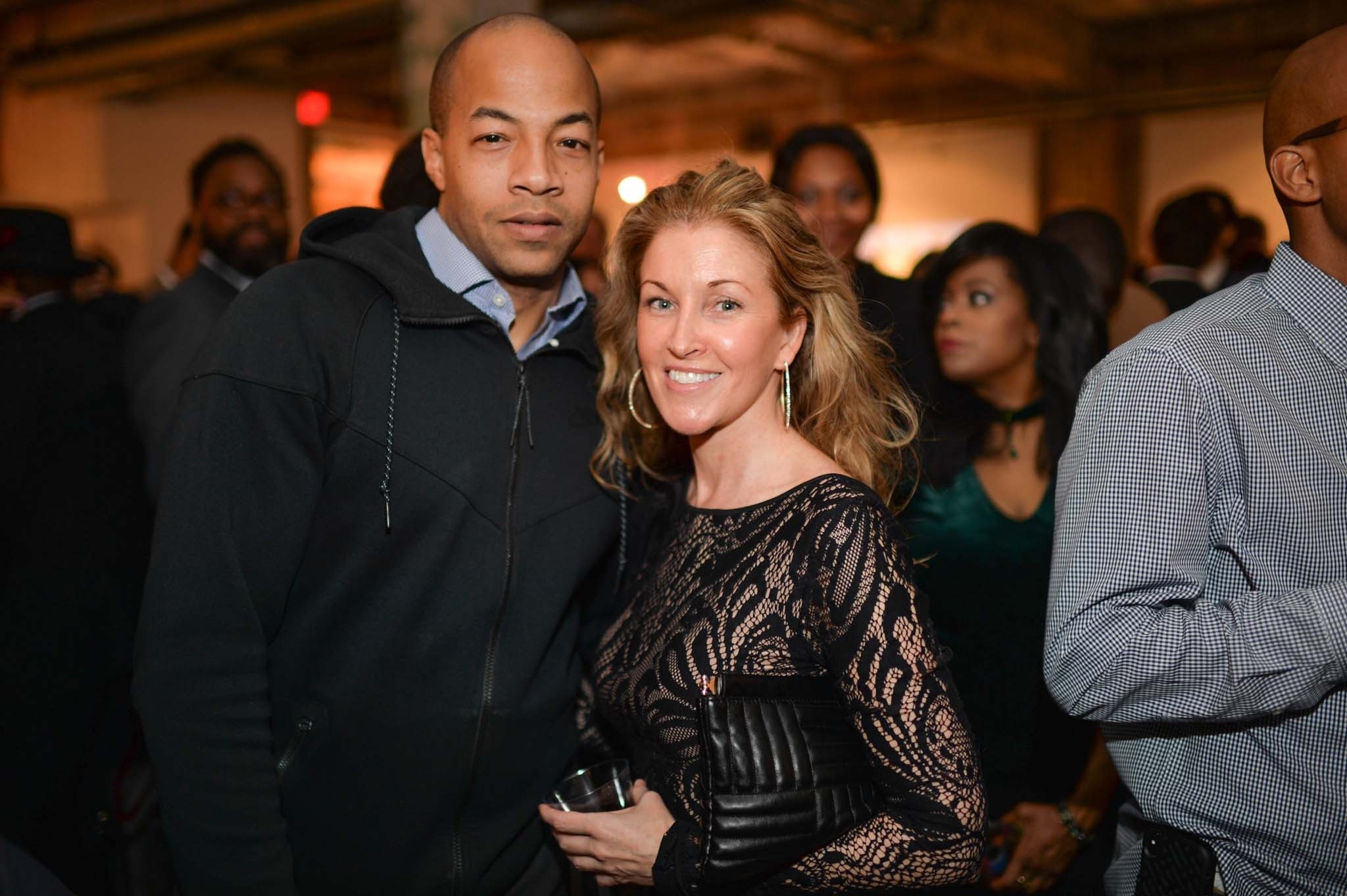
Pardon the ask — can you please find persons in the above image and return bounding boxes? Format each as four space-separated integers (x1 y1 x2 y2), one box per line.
762 117 949 467
878 219 1114 895
127 132 291 493
122 9 620 893
1037 179 1273 353
0 199 197 895
1040 23 1345 896
534 152 989 896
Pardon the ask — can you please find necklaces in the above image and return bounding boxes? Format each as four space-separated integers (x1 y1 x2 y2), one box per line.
988 394 1042 460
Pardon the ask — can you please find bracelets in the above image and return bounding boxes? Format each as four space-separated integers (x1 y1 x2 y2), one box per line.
1057 801 1093 843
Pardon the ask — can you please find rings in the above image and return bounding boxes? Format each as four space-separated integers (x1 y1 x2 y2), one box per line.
1016 874 1025 884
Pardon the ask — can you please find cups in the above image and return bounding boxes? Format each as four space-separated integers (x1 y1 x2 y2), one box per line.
556 756 637 838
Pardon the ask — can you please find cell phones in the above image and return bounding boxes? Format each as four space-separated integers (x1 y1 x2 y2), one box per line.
1134 823 1216 895
